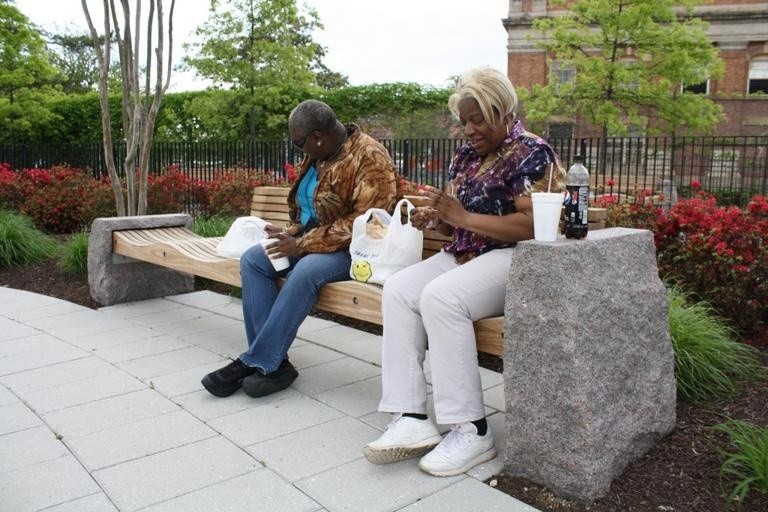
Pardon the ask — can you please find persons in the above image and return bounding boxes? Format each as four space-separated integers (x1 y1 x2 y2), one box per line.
200 98 400 399
362 65 567 477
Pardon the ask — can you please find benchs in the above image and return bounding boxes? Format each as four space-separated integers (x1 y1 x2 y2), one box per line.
111 187 608 356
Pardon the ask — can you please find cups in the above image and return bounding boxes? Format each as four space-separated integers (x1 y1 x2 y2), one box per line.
530 192 565 243
260 237 290 272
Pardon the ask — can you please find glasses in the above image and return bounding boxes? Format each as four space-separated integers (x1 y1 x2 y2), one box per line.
293 136 307 150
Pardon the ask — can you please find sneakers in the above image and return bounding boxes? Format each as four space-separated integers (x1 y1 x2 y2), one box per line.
201 358 257 397
363 413 442 465
419 421 496 476
243 359 298 398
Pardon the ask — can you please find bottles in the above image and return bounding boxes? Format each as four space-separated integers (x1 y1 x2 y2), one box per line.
565 155 590 238
